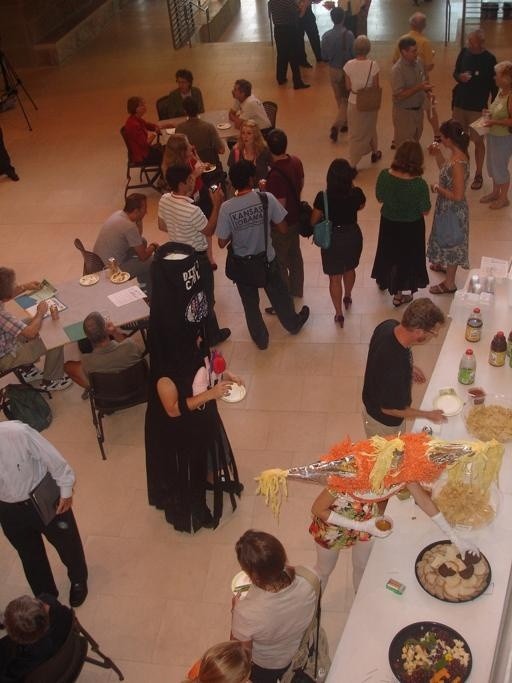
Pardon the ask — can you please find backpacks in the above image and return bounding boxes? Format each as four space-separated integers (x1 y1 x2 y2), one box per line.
0 383 54 433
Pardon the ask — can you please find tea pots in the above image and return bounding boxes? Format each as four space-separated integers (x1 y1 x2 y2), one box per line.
103 257 119 279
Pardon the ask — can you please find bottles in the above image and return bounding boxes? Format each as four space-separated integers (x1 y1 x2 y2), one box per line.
457 349 476 384
108 257 117 273
465 307 482 342
210 184 217 192
50 304 58 320
488 331 507 367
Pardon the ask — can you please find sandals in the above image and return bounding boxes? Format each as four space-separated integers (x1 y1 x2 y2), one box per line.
391 139 397 149
479 192 500 203
490 196 511 209
433 135 442 144
393 293 414 308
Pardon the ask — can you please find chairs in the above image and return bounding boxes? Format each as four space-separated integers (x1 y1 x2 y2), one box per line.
21 605 125 682
261 100 278 139
155 95 170 121
88 357 151 461
119 125 165 200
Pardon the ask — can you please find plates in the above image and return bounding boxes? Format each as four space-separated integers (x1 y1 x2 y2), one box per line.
414 538 492 604
79 274 99 286
389 621 472 683
219 382 246 403
433 394 463 417
216 122 231 129
203 166 216 173
111 271 130 284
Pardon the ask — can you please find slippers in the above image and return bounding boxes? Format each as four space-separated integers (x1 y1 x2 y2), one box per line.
429 261 447 274
429 282 458 295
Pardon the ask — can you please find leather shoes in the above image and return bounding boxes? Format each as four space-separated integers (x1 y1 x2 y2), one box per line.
68 577 88 608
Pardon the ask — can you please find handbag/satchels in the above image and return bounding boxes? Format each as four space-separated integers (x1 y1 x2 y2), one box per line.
266 166 314 239
224 192 271 289
280 566 332 683
313 189 334 249
339 29 351 99
354 60 383 111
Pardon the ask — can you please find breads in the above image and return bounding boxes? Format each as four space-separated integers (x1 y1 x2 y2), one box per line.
417 544 488 601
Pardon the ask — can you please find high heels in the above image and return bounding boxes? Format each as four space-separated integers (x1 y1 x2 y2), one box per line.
334 313 345 328
342 296 353 310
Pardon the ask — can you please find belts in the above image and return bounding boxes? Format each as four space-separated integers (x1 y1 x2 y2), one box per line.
406 106 422 111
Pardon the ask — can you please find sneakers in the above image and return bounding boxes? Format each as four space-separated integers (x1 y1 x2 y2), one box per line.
370 150 383 163
470 174 486 191
209 326 232 348
21 366 45 382
300 60 313 69
329 125 339 142
7 168 21 182
41 375 74 392
294 82 311 89
290 305 311 335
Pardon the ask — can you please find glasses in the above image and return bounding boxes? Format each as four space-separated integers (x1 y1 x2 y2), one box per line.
425 328 439 338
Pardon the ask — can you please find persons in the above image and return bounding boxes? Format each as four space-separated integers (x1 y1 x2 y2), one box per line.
62 311 144 401
227 118 272 188
216 158 309 350
337 0 367 40
159 132 217 272
91 192 157 305
343 34 382 179
185 639 254 683
370 141 429 307
228 528 322 683
160 69 205 119
0 266 73 392
360 297 449 438
393 12 439 129
227 78 272 137
448 30 500 192
294 0 331 70
267 1 311 90
174 95 228 201
257 129 305 316
1 128 21 182
157 162 231 349
479 60 512 210
123 96 162 168
310 159 367 329
144 322 246 533
308 432 481 597
389 34 435 151
1 591 73 683
0 420 88 608
321 8 356 142
428 119 471 295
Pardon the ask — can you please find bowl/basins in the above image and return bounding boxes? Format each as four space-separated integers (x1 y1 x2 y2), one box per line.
460 392 512 444
466 386 487 405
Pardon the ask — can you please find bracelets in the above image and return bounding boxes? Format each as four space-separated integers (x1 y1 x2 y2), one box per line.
193 394 207 413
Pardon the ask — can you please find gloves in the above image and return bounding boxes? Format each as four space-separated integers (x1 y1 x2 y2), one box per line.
429 510 481 562
325 510 394 537
